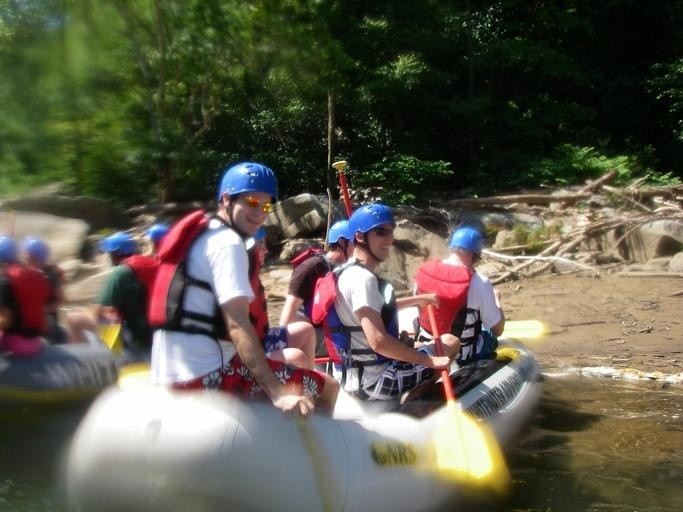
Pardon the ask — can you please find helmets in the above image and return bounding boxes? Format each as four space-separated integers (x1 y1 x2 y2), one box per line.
327 219 351 245
0 236 19 263
449 227 482 256
216 163 279 203
99 232 136 256
348 204 396 238
24 238 49 263
146 225 169 241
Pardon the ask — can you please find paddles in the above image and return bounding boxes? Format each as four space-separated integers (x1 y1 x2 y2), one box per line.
426 304 492 477
497 320 544 340
96 323 121 350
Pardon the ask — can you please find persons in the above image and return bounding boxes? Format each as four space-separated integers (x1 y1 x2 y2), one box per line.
411 225 506 363
17 237 73 344
142 222 170 253
147 160 342 423
0 233 51 358
61 228 153 361
318 201 463 404
276 217 358 360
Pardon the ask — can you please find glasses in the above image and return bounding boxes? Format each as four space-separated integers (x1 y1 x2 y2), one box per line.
372 226 393 237
240 195 271 214
